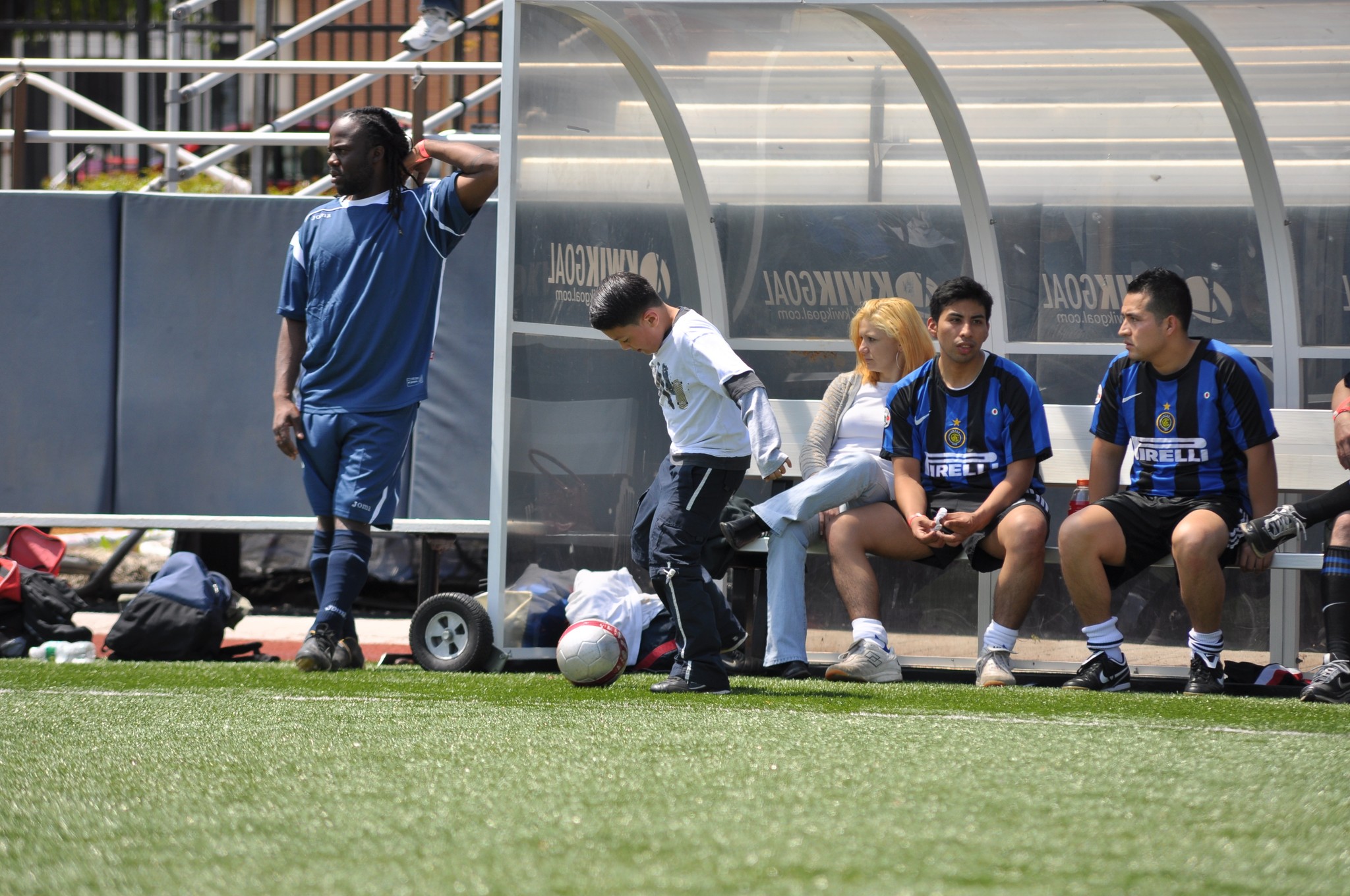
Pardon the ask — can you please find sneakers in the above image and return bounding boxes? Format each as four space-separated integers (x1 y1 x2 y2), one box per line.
650 677 731 695
825 637 903 683
1301 652 1350 705
975 646 1018 686
295 625 336 672
1239 503 1311 558
1184 652 1225 697
1062 652 1130 692
720 626 748 651
719 649 777 677
332 637 364 670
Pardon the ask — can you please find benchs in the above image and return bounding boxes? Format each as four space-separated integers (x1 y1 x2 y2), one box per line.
515 47 1350 207
737 396 1350 672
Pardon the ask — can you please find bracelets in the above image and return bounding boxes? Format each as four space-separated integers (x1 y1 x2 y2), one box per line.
415 138 432 163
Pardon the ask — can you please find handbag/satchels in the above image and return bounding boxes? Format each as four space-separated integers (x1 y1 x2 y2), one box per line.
640 610 677 673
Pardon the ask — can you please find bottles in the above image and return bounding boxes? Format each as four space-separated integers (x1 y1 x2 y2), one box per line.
1068 479 1089 516
28 640 96 664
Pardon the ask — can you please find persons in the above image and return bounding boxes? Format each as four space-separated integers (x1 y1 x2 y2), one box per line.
1237 368 1350 702
397 0 464 50
720 298 935 679
1058 267 1280 695
271 107 501 674
822 277 1054 684
588 272 792 696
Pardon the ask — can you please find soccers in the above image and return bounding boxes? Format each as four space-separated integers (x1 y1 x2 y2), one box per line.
556 618 630 688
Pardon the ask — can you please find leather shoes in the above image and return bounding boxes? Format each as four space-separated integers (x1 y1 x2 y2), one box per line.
720 515 760 549
783 659 810 680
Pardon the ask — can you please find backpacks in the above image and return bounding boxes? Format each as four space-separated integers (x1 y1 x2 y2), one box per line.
102 551 254 662
0 525 93 658
